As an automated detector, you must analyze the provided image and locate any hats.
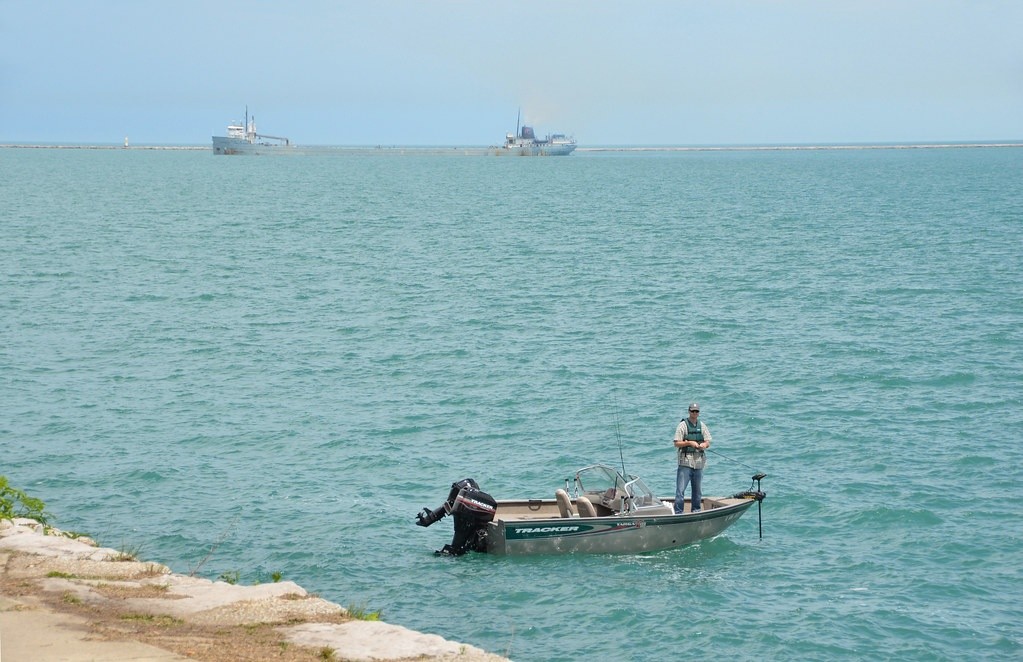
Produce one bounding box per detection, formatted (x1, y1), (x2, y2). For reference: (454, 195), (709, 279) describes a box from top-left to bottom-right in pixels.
(688, 402), (700, 412)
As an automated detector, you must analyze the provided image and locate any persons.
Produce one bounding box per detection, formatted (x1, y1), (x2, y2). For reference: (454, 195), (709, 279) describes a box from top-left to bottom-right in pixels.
(673, 403), (712, 516)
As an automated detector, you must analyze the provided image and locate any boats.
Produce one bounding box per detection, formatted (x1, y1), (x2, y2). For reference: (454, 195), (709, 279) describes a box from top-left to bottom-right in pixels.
(210, 103), (292, 160)
(459, 105), (577, 158)
(413, 460), (768, 565)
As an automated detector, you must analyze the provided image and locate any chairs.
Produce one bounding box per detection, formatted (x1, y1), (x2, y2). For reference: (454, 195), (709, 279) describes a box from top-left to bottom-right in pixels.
(556, 488), (574, 518)
(576, 497), (596, 518)
